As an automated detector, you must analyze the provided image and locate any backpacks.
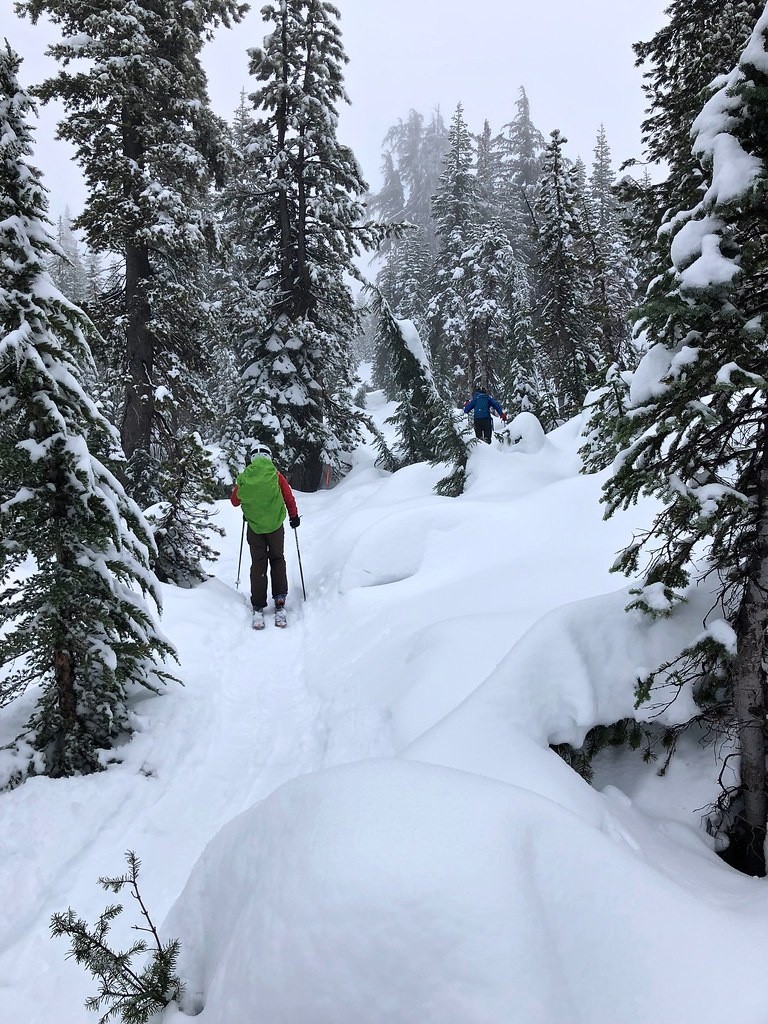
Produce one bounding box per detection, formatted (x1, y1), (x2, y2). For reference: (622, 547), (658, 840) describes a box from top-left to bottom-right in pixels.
(237, 456), (286, 534)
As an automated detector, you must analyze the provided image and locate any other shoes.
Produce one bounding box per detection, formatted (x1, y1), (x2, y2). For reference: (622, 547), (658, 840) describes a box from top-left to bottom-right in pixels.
(253, 604), (263, 613)
(275, 594), (285, 609)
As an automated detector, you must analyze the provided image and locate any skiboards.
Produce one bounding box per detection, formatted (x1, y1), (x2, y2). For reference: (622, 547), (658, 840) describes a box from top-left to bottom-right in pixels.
(252, 608), (286, 631)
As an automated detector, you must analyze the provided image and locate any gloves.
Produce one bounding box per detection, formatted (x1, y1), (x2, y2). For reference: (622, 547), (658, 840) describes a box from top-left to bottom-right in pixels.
(465, 400), (470, 406)
(289, 514), (300, 528)
(500, 414), (506, 421)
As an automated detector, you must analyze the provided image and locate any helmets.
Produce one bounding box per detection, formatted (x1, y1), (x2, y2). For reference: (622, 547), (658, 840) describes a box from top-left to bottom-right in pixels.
(476, 387), (486, 393)
(250, 445), (272, 463)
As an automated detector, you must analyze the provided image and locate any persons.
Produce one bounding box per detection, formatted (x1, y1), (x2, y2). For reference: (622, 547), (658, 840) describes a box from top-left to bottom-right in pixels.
(231, 444), (300, 629)
(464, 387), (506, 444)
